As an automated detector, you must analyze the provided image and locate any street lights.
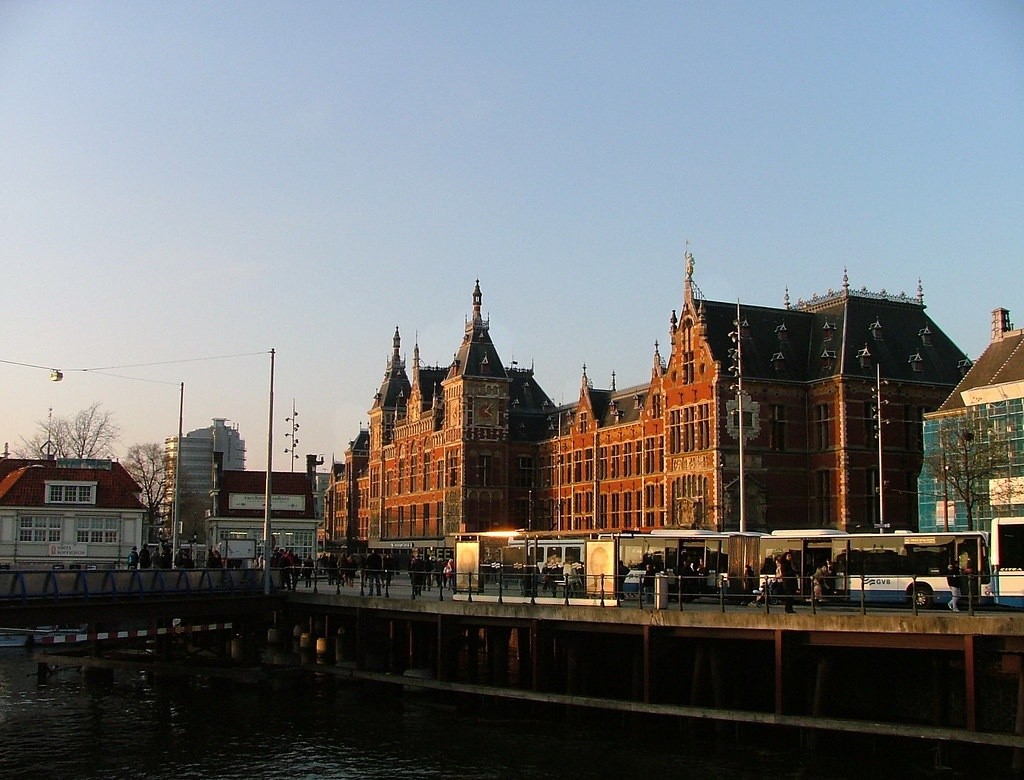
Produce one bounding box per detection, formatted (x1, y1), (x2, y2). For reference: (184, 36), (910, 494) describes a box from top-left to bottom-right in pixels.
(872, 364), (891, 534)
(284, 398), (299, 471)
(728, 298), (749, 533)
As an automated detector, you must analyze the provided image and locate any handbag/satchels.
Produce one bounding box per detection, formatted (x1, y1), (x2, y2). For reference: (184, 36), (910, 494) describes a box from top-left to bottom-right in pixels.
(446, 563), (452, 577)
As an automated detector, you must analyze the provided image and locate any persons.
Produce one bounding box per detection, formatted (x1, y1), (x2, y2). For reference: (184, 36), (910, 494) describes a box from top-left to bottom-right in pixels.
(526, 556), (540, 594)
(678, 558), (704, 603)
(947, 562), (962, 612)
(964, 561), (978, 595)
(484, 552), (501, 582)
(814, 559), (833, 599)
(685, 251), (695, 278)
(227, 559), (243, 568)
(741, 565), (756, 604)
(776, 554), (804, 613)
(563, 557), (578, 598)
(580, 564), (584, 586)
(620, 560), (630, 601)
(634, 564), (659, 604)
(408, 555), (454, 596)
(542, 563), (560, 597)
(270, 548), (395, 596)
(259, 557), (263, 569)
(207, 549), (222, 568)
(128, 543), (195, 569)
(638, 563), (644, 569)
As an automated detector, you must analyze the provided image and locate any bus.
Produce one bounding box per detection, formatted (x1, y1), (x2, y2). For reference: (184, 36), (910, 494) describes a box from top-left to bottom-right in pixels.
(990, 517), (1024, 608)
(598, 529), (772, 599)
(759, 529), (993, 610)
(508, 540), (585, 585)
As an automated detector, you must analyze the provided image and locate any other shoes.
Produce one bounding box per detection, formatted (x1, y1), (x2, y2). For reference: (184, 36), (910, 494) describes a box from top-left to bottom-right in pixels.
(784, 609), (796, 613)
(953, 609), (960, 612)
(948, 603), (952, 609)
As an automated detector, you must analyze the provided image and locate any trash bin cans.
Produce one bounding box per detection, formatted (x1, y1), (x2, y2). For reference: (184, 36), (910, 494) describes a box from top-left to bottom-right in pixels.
(654, 570), (669, 610)
